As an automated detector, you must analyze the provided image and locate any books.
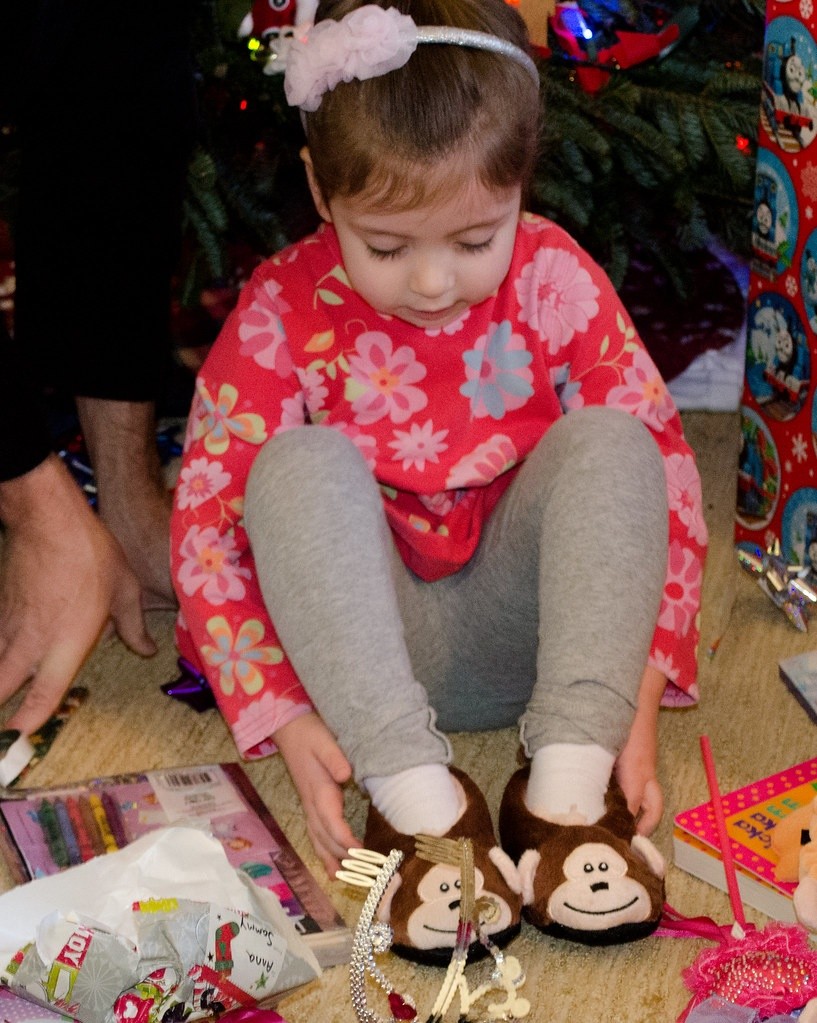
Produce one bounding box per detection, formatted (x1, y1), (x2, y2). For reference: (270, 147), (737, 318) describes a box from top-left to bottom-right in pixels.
(671, 759), (817, 921)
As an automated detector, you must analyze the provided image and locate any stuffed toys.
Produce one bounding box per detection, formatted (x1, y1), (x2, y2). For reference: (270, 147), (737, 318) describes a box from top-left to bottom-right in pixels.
(773, 796), (817, 931)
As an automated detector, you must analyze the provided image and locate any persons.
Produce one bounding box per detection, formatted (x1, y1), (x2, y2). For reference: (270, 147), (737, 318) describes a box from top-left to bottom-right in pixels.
(171, 0), (708, 968)
(0, 0), (198, 734)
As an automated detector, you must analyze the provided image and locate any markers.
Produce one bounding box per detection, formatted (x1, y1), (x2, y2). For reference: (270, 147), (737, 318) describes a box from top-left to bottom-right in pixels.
(36, 790), (126, 870)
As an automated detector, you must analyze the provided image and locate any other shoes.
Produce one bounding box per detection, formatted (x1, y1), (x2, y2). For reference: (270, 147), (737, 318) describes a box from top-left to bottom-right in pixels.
(364, 764), (521, 969)
(498, 757), (666, 946)
